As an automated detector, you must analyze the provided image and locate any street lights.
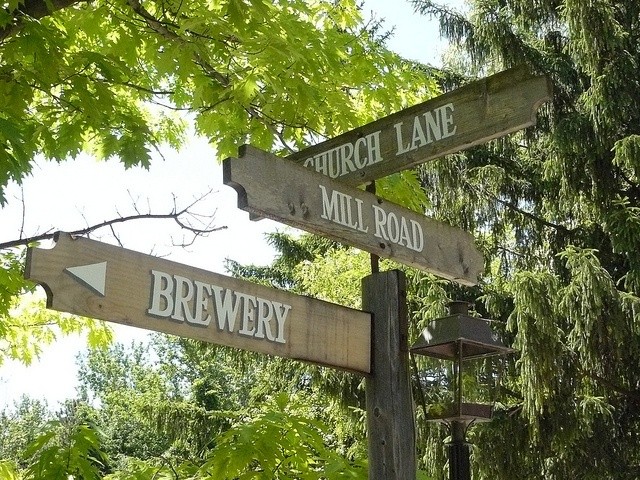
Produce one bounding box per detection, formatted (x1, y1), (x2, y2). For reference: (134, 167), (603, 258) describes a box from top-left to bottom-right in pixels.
(410, 302), (518, 480)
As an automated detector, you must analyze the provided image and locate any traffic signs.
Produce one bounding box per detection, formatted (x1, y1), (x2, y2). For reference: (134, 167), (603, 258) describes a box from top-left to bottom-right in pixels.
(223, 144), (484, 288)
(249, 64), (553, 221)
(25, 231), (371, 374)
